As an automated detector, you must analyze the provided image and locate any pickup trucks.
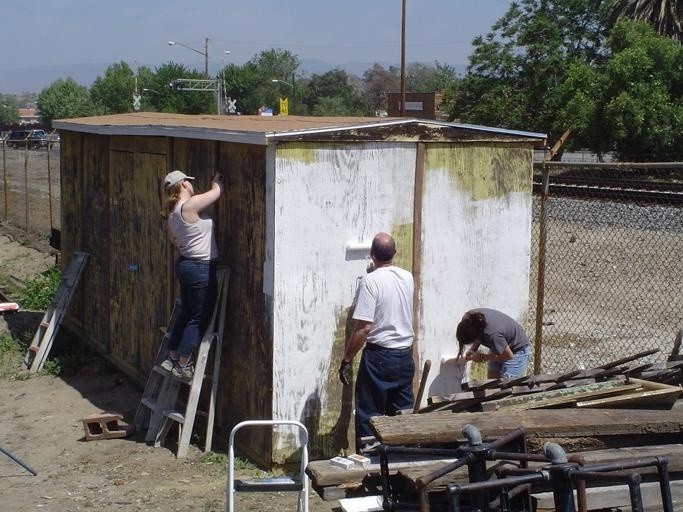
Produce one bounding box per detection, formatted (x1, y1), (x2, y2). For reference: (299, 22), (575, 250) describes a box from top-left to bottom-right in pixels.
(10, 130), (60, 150)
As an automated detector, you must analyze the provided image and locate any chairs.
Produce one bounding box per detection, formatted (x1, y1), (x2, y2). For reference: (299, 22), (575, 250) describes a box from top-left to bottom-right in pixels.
(226, 419), (309, 512)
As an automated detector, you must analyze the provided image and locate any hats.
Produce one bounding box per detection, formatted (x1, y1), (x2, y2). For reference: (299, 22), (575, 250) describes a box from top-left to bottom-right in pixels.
(163, 171), (195, 191)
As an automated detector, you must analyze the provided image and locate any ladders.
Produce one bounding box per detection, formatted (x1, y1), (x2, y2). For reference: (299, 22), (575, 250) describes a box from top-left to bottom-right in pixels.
(21, 251), (89, 374)
(132, 268), (231, 459)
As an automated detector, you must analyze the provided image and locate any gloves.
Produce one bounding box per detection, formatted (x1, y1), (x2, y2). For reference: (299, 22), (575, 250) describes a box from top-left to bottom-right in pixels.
(340, 359), (350, 386)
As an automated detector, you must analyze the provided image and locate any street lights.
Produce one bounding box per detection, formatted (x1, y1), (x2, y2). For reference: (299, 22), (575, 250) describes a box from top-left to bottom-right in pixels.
(143, 88), (160, 112)
(168, 37), (209, 76)
(272, 73), (296, 116)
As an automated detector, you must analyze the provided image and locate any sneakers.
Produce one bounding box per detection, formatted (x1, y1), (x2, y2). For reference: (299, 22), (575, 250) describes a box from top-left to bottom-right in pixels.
(161, 357), (207, 377)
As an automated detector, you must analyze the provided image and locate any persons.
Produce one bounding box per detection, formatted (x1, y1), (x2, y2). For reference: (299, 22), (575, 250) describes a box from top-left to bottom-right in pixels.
(159, 170), (224, 382)
(337, 233), (414, 453)
(455, 308), (530, 380)
